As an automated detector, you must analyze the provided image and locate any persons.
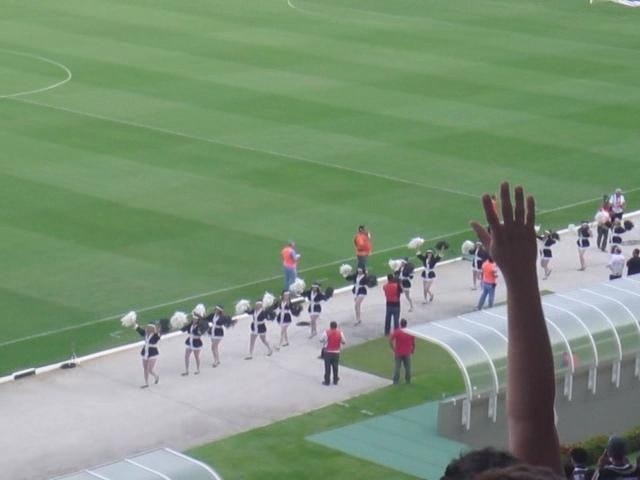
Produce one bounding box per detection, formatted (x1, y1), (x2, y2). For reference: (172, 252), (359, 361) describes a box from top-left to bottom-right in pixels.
(131, 320), (160, 389)
(243, 300), (273, 359)
(319, 321), (346, 385)
(206, 305), (231, 368)
(352, 225), (370, 269)
(298, 282), (329, 338)
(440, 178), (568, 480)
(380, 187), (640, 337)
(179, 315), (201, 375)
(591, 433), (635, 480)
(270, 289), (303, 350)
(346, 265), (370, 326)
(567, 447), (594, 480)
(281, 240), (301, 289)
(387, 319), (416, 385)
